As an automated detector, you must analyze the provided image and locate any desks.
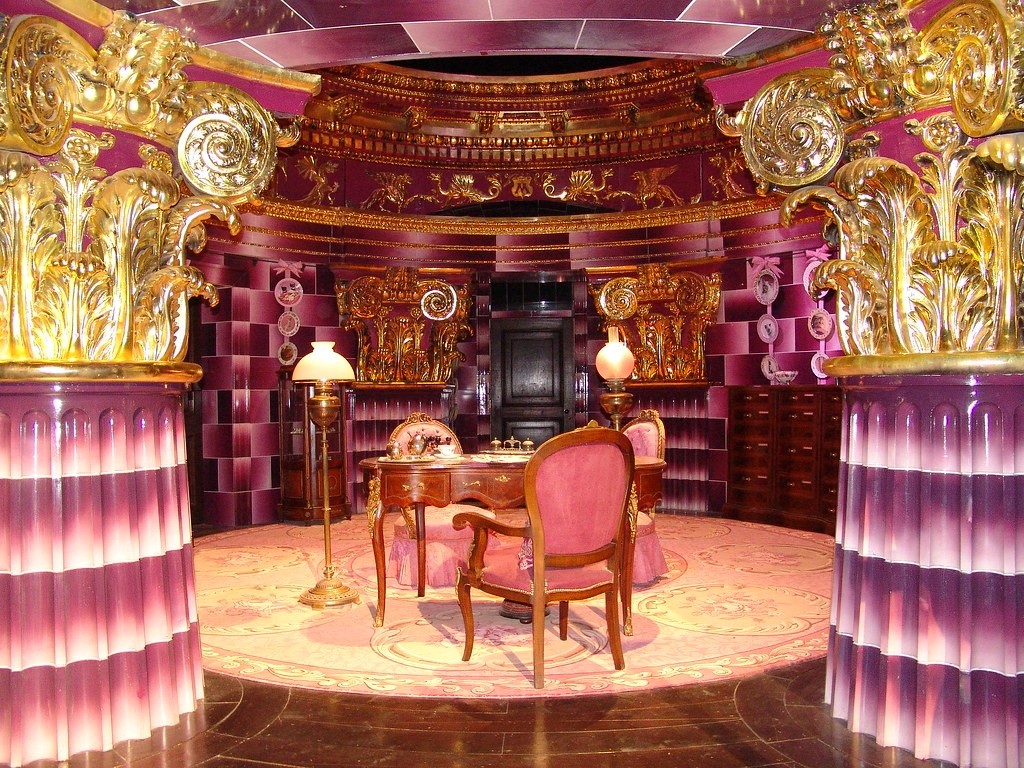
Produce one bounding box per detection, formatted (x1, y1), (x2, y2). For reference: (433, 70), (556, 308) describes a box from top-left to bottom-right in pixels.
(358, 453), (669, 637)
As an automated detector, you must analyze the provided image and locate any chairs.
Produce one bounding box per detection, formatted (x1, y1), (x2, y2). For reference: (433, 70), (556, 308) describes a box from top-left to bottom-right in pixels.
(451, 420), (634, 690)
(385, 412), (502, 588)
(619, 409), (668, 585)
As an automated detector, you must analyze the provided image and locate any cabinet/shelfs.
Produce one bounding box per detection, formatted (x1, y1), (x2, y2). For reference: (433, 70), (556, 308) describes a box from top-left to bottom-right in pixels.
(729, 384), (843, 538)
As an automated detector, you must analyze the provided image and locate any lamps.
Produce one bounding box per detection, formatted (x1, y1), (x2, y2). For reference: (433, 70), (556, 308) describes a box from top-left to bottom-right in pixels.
(596, 326), (634, 432)
(292, 340), (361, 612)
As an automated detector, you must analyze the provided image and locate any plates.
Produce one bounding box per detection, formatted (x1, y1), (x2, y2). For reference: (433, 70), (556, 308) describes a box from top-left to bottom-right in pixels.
(434, 454), (460, 459)
(757, 314), (778, 343)
(761, 355), (779, 380)
(803, 261), (829, 299)
(811, 353), (832, 379)
(808, 308), (832, 339)
(278, 342), (298, 365)
(274, 278), (304, 307)
(278, 311), (300, 337)
(752, 269), (779, 305)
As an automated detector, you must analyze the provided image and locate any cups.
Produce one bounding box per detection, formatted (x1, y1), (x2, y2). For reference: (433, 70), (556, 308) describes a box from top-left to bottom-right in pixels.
(438, 445), (456, 456)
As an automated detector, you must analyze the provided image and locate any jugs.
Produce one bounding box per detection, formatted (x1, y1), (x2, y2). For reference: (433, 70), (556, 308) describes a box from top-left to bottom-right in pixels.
(406, 432), (428, 457)
(386, 442), (404, 460)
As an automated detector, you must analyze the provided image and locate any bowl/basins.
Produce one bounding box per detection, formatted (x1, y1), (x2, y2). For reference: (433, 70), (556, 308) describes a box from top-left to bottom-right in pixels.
(773, 370), (798, 384)
